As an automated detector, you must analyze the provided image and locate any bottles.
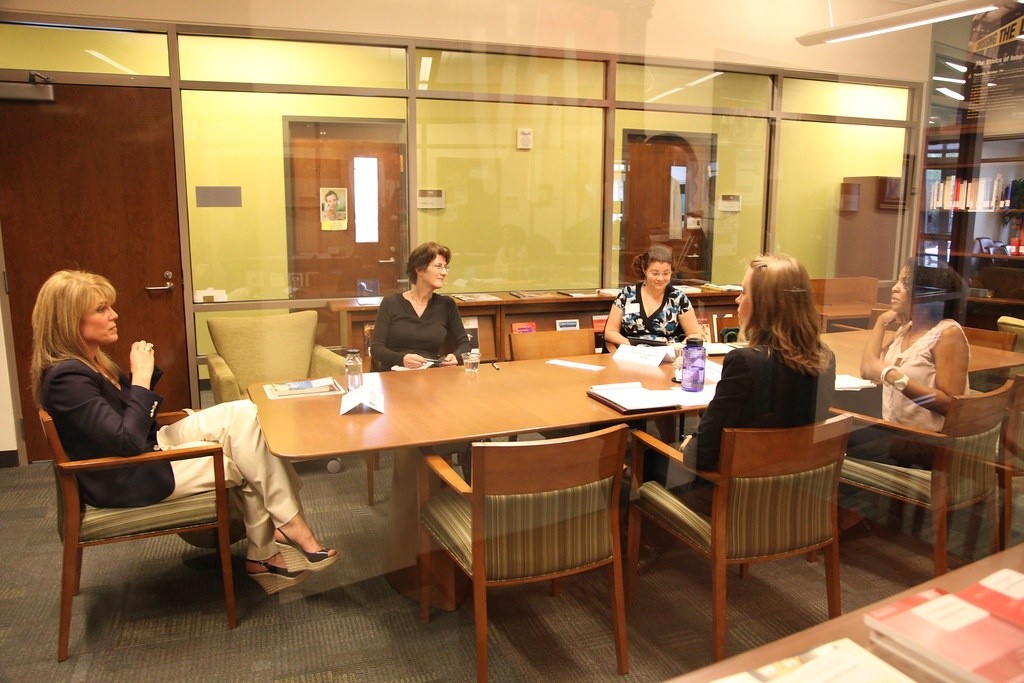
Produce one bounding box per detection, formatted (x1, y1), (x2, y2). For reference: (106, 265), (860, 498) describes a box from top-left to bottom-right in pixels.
(344, 349), (363, 393)
(682, 337), (706, 391)
(327, 458), (342, 473)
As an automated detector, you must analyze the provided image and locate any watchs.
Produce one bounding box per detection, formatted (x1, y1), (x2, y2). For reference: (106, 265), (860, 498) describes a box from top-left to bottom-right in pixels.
(893, 375), (909, 391)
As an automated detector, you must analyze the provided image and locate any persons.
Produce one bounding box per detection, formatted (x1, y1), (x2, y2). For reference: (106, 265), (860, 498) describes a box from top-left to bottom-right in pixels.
(368, 241), (492, 486)
(602, 245), (705, 444)
(323, 190), (346, 221)
(838, 258), (972, 543)
(28, 271), (339, 595)
(636, 255), (835, 579)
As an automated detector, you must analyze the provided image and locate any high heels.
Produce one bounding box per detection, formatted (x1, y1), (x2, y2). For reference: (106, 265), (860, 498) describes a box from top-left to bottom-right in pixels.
(274, 528), (340, 573)
(244, 558), (313, 595)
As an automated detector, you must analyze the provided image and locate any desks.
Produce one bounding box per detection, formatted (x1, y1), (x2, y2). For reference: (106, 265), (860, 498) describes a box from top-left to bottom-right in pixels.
(247, 332), (1024, 629)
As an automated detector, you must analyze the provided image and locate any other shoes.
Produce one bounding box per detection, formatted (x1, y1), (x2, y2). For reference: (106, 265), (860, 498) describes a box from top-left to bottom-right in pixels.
(635, 526), (675, 575)
(838, 516), (874, 540)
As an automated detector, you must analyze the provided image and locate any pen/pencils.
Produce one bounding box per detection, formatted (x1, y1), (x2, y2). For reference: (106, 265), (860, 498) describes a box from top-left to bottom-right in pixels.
(491, 360), (499, 370)
(884, 319), (896, 326)
(424, 359), (448, 362)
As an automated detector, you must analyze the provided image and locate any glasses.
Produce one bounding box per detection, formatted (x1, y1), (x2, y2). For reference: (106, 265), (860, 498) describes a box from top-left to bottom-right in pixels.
(427, 263), (448, 270)
(646, 271), (673, 276)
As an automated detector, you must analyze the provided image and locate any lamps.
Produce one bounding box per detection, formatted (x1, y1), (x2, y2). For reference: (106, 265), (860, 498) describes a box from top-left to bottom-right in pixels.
(794, 0), (1003, 47)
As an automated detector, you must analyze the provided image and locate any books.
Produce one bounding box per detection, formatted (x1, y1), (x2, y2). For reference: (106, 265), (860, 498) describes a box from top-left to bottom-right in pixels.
(926, 171), (1009, 212)
(587, 390), (682, 415)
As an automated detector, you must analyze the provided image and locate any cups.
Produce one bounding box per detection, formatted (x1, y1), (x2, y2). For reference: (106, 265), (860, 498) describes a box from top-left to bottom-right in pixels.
(675, 347), (684, 381)
(461, 353), (481, 372)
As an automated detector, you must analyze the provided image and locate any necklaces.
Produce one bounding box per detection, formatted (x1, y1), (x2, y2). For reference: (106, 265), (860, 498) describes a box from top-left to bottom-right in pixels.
(410, 290), (428, 307)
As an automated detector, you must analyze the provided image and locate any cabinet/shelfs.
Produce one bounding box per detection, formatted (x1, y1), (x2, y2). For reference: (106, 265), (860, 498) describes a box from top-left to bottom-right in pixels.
(914, 155), (1024, 296)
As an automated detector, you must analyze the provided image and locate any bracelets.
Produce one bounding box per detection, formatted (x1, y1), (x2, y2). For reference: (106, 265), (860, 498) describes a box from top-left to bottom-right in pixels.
(880, 367), (896, 386)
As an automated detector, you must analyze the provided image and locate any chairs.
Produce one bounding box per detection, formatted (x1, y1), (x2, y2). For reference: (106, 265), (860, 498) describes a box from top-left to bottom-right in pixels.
(827, 375), (1024, 576)
(206, 310), (347, 474)
(509, 327), (596, 440)
(998, 317), (1024, 460)
(625, 410), (855, 663)
(961, 326), (1017, 379)
(419, 423), (630, 683)
(38, 407), (237, 661)
